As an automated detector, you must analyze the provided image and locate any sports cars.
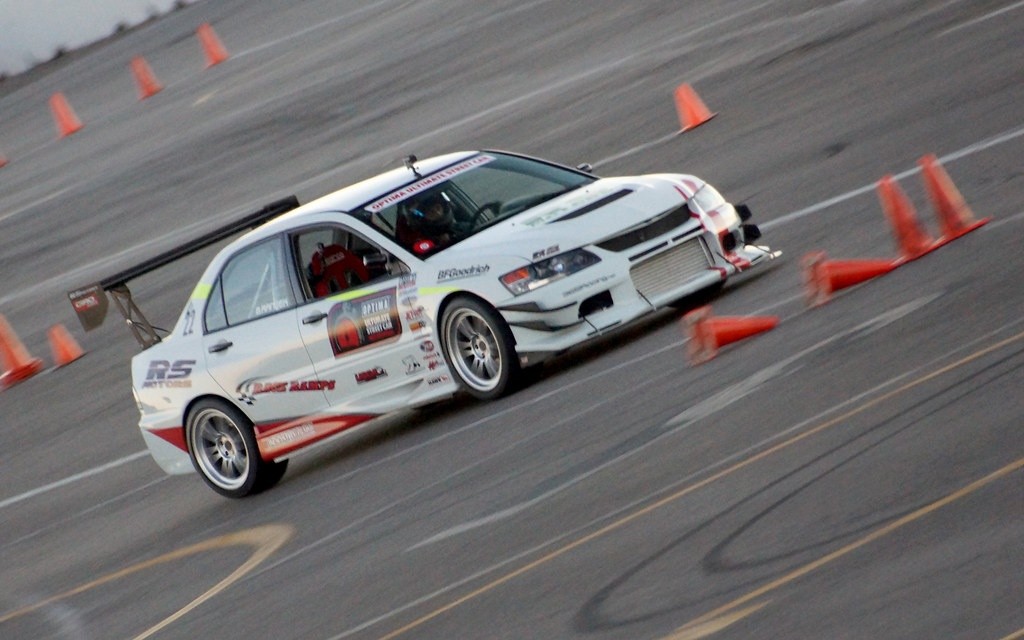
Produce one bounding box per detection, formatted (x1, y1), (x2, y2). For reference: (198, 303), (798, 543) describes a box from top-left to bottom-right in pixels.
(94, 147), (778, 503)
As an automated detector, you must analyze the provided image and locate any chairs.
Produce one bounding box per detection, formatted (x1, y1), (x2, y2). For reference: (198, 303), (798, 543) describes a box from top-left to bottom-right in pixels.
(306, 243), (372, 300)
(392, 201), (435, 254)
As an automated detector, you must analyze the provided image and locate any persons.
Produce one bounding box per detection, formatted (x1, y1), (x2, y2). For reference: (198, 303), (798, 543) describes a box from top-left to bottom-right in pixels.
(402, 191), (476, 254)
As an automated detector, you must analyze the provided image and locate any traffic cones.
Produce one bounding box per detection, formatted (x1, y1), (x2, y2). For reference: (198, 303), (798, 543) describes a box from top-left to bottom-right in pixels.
(199, 20), (228, 69)
(871, 178), (942, 270)
(672, 83), (717, 139)
(0, 315), (47, 384)
(912, 150), (989, 245)
(44, 325), (89, 372)
(49, 93), (84, 141)
(132, 59), (165, 104)
(682, 305), (781, 367)
(801, 247), (895, 300)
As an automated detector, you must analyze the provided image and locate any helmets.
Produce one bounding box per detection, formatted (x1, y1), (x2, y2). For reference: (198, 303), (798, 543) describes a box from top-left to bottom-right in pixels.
(402, 189), (453, 235)
